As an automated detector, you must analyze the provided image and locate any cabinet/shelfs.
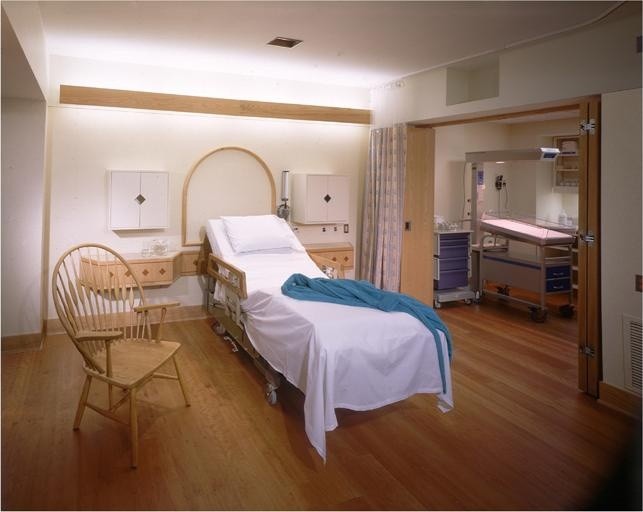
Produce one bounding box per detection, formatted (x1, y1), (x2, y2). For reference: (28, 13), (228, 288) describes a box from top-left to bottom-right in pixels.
(292, 173), (351, 227)
(105, 169), (171, 230)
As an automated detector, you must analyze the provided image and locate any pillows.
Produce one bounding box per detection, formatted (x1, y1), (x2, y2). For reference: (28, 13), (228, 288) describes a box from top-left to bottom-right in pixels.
(222, 215), (299, 253)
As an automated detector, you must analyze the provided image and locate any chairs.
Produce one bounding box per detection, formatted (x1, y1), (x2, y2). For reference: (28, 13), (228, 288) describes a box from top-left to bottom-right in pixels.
(52, 242), (191, 471)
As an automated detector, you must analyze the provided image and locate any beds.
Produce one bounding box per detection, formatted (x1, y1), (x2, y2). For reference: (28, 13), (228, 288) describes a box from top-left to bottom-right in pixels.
(194, 218), (448, 408)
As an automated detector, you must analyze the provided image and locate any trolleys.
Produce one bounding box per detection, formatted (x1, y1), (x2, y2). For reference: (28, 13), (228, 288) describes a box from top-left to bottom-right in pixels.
(433, 208), (576, 322)
